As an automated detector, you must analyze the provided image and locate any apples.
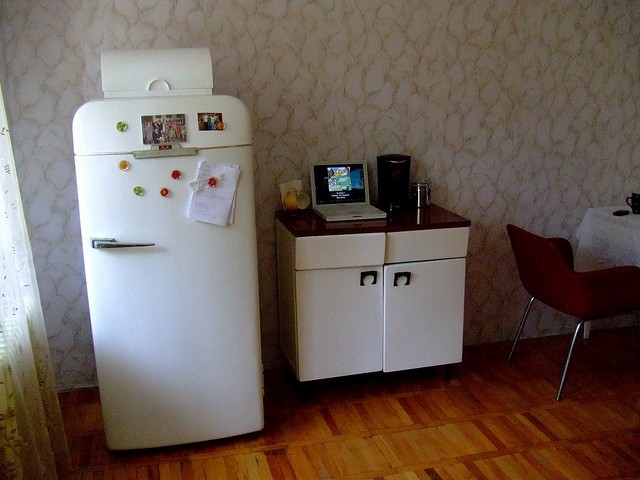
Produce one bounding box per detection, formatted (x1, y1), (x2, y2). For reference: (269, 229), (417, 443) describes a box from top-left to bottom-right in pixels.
(286, 196), (297, 211)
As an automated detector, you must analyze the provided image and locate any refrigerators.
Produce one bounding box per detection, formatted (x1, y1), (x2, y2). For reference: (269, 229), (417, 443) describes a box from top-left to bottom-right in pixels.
(71, 95), (264, 451)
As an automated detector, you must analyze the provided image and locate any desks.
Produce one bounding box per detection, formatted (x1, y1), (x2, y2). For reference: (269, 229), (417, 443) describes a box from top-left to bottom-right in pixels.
(584, 204), (640, 339)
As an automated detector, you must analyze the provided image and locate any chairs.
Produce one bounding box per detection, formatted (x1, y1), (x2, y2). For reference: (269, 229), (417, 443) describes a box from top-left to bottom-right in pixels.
(505, 224), (640, 402)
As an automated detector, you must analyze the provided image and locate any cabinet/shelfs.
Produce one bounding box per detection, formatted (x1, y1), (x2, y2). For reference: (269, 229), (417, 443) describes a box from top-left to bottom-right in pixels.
(274, 202), (471, 400)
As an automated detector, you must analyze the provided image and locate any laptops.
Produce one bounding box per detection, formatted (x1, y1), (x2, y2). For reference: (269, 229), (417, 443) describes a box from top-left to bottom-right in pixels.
(309, 160), (388, 223)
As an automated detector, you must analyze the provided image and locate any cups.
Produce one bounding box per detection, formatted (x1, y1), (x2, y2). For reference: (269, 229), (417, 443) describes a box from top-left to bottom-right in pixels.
(626, 191), (639, 214)
(411, 179), (432, 208)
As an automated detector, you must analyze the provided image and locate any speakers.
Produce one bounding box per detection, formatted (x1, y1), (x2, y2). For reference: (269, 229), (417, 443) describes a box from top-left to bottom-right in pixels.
(377, 155), (412, 215)
